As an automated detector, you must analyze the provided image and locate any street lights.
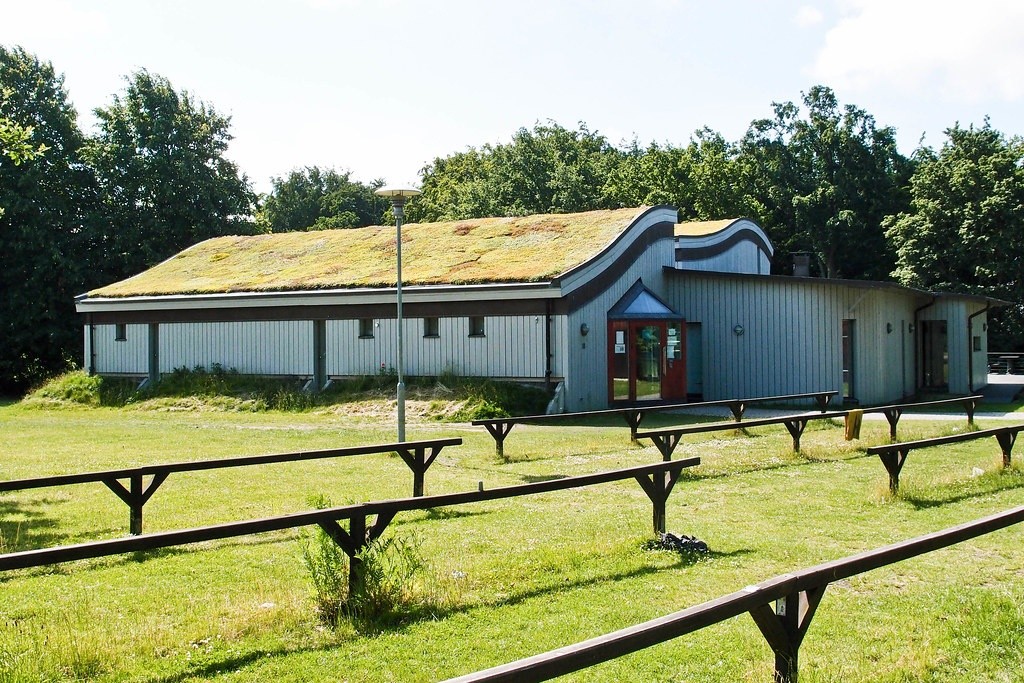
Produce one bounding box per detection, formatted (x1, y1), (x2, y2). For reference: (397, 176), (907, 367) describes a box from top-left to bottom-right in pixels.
(375, 182), (422, 442)
